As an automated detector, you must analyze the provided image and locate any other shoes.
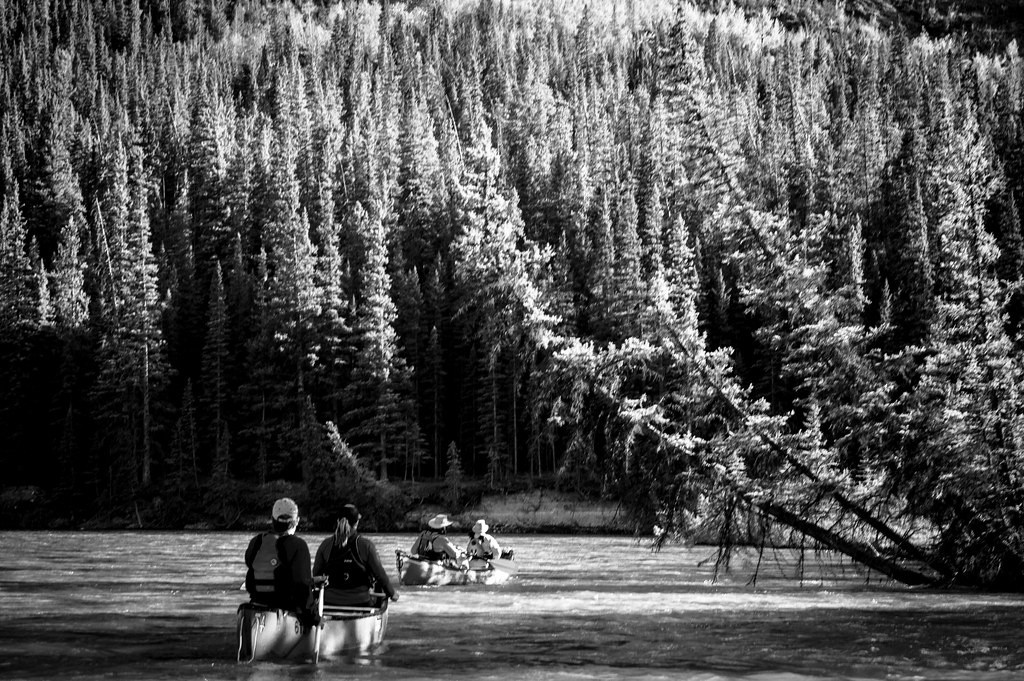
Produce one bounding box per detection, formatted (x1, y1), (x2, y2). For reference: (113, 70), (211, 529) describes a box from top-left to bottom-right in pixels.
(503, 550), (513, 560)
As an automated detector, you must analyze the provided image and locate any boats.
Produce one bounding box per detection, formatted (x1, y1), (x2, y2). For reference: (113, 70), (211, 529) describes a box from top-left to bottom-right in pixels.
(232, 593), (389, 665)
(394, 548), (496, 587)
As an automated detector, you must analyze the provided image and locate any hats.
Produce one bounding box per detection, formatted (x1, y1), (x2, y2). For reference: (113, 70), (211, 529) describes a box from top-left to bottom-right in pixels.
(273, 498), (298, 522)
(339, 503), (359, 527)
(472, 519), (489, 534)
(428, 514), (453, 528)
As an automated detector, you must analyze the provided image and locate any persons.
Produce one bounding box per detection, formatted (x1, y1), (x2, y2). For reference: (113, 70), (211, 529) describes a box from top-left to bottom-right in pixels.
(312, 504), (399, 607)
(467, 519), (512, 560)
(411, 514), (460, 561)
(245, 498), (311, 609)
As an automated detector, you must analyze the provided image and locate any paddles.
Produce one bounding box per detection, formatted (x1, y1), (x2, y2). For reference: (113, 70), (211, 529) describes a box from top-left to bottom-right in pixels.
(463, 553), (517, 574)
(314, 582), (326, 664)
